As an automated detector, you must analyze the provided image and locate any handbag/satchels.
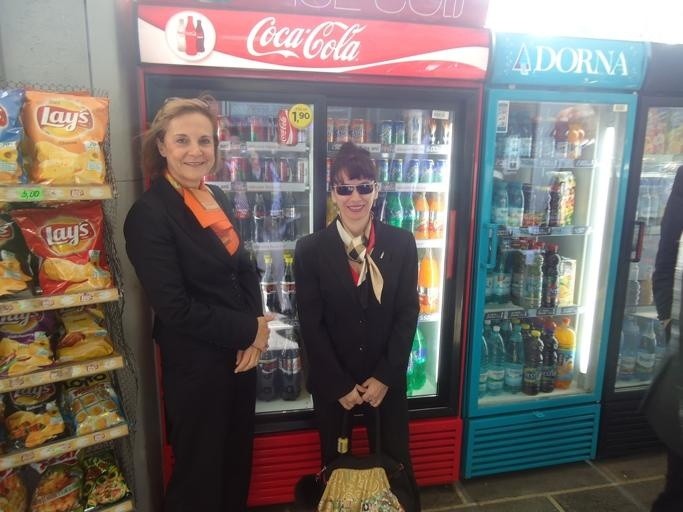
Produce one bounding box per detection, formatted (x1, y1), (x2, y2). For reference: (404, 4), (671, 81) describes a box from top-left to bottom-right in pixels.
(638, 343), (682, 458)
(294, 455), (419, 512)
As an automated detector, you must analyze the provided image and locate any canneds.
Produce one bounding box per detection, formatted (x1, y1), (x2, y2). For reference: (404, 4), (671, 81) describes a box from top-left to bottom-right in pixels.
(203, 108), (309, 186)
(326, 109), (452, 183)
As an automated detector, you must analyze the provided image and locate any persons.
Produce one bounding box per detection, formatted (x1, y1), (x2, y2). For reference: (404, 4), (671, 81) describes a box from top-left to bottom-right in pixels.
(639, 166), (683, 512)
(123, 92), (276, 511)
(292, 141), (421, 512)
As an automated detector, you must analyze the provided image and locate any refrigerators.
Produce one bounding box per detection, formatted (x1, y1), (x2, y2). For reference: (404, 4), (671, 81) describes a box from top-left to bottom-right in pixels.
(460, 31), (648, 482)
(126, 1), (491, 504)
(589, 43), (682, 461)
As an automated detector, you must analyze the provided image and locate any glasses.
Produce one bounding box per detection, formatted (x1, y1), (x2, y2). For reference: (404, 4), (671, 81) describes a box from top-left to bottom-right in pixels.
(161, 96), (210, 109)
(330, 182), (376, 196)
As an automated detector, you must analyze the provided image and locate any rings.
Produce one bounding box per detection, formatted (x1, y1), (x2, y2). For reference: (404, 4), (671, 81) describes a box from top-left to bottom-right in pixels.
(369, 396), (372, 401)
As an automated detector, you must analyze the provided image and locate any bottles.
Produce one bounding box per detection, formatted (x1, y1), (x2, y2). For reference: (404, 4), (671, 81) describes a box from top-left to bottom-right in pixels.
(608, 118), (681, 392)
(475, 102), (599, 399)
(326, 193), (454, 401)
(184, 16), (196, 56)
(201, 189), (313, 405)
(176, 20), (185, 52)
(195, 20), (204, 53)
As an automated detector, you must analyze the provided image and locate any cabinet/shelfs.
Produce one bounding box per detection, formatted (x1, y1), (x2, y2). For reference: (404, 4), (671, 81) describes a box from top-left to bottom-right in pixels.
(0, 185), (134, 512)
